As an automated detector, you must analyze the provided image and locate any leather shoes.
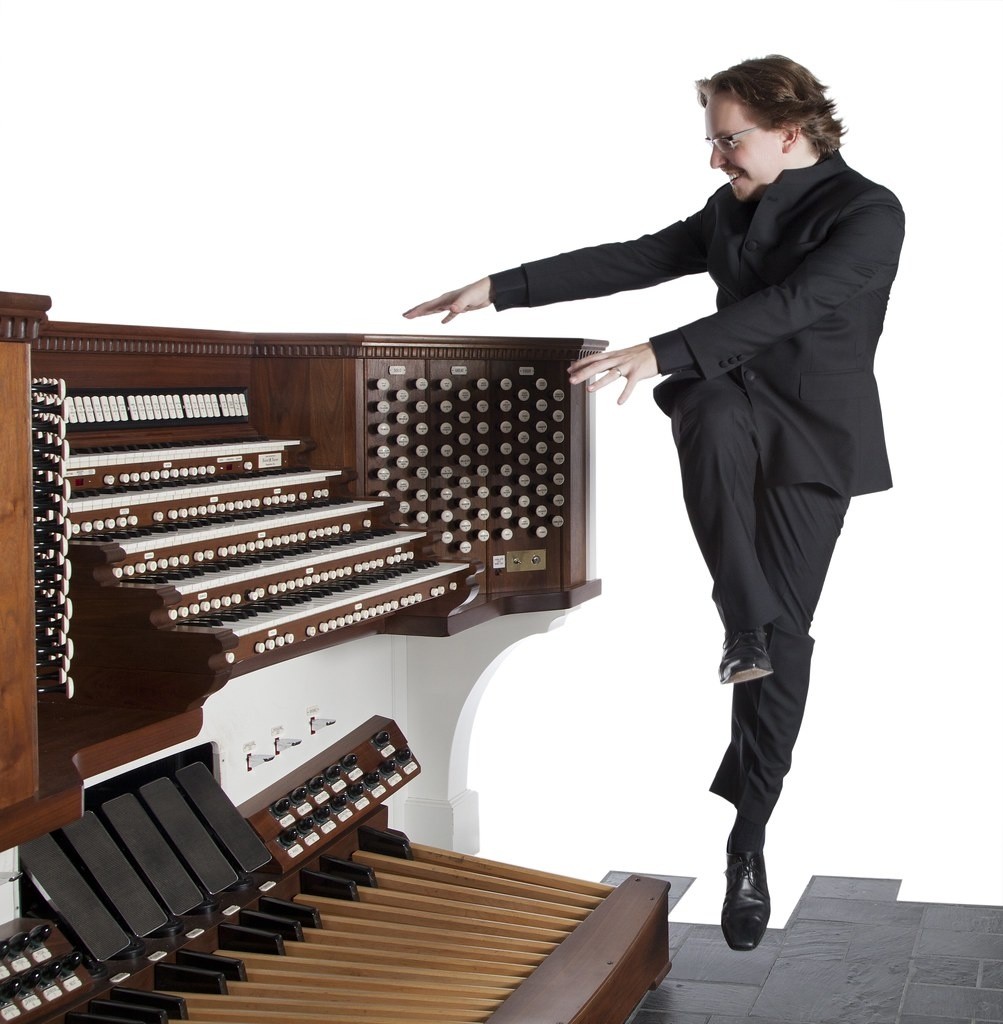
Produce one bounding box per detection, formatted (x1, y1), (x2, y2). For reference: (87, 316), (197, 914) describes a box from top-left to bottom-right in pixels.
(717, 625), (775, 684)
(720, 836), (771, 952)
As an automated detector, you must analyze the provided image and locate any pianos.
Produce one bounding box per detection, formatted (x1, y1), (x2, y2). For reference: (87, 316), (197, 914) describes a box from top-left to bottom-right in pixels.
(0, 290), (672, 1024)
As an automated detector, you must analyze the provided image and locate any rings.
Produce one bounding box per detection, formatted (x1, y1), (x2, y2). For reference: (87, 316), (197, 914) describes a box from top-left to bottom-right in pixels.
(614, 367), (623, 376)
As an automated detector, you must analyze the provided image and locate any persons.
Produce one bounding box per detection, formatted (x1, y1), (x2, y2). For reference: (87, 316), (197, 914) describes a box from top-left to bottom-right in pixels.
(401, 55), (904, 952)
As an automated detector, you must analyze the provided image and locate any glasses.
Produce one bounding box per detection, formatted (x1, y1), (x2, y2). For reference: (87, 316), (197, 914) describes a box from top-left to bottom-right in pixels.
(704, 126), (762, 153)
